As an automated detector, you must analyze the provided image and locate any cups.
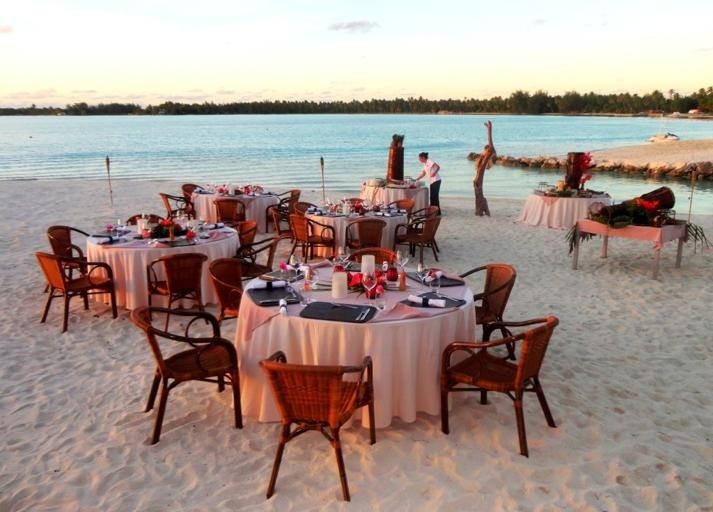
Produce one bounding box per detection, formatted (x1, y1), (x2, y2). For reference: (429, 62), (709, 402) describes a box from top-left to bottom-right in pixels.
(363, 255), (375, 276)
(416, 261), (430, 294)
(332, 273), (345, 300)
(292, 254), (304, 289)
(306, 270), (318, 304)
(338, 245), (351, 270)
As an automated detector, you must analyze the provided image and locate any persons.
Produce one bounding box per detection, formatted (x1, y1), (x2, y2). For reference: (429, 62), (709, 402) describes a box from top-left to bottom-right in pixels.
(416, 151), (442, 216)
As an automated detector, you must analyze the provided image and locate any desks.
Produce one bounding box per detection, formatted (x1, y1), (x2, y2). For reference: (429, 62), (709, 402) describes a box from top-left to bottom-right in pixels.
(571, 219), (687, 280)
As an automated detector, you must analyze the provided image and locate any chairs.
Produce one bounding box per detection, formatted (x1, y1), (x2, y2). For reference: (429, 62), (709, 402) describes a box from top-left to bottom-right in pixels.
(258, 350), (376, 502)
(233, 220), (258, 251)
(146, 252), (209, 334)
(393, 216), (443, 268)
(292, 200), (318, 216)
(181, 182), (205, 199)
(440, 316), (560, 457)
(289, 214), (336, 263)
(235, 237), (279, 277)
(268, 206), (294, 240)
(408, 205), (440, 254)
(34, 250), (117, 330)
(344, 218), (387, 254)
(387, 199), (416, 220)
(213, 198), (246, 225)
(129, 303), (244, 445)
(158, 192), (197, 221)
(453, 262), (517, 361)
(347, 247), (397, 264)
(264, 189), (301, 234)
(347, 196), (365, 204)
(127, 214), (166, 225)
(48, 224), (114, 282)
(207, 257), (244, 325)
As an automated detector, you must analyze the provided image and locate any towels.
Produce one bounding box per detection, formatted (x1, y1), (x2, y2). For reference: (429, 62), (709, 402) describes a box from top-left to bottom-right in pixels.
(406, 293), (447, 312)
(95, 235), (119, 244)
(314, 210), (327, 216)
(248, 190), (261, 198)
(249, 279), (288, 291)
(278, 297), (288, 316)
(376, 211), (390, 216)
(390, 209), (406, 213)
(425, 270), (444, 284)
(204, 222), (227, 230)
(307, 207), (319, 211)
